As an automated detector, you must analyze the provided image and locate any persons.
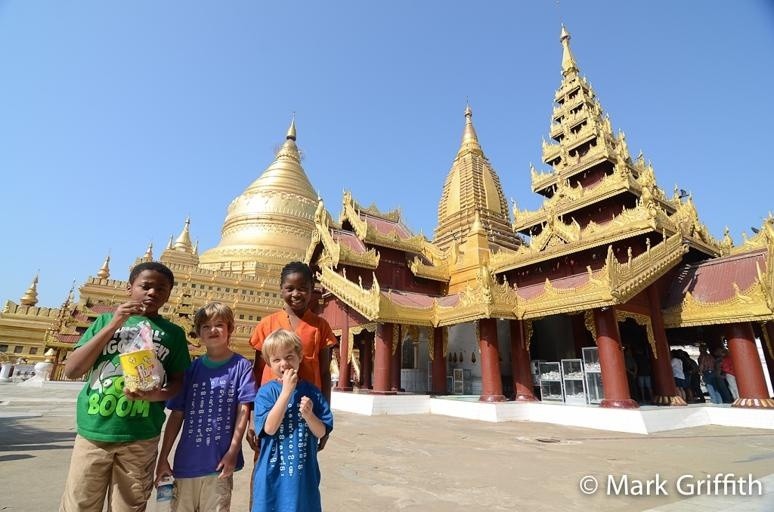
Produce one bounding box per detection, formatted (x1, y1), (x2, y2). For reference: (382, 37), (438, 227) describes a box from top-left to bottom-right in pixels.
(58, 262), (191, 512)
(669, 343), (740, 405)
(622, 346), (654, 404)
(154, 302), (258, 512)
(250, 327), (333, 511)
(246, 261), (340, 512)
